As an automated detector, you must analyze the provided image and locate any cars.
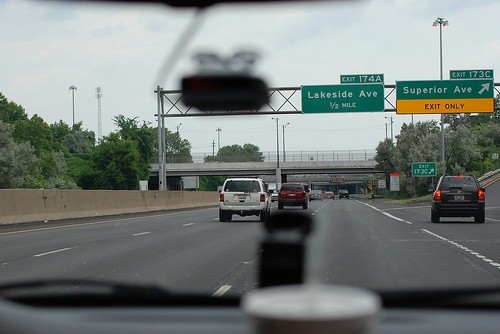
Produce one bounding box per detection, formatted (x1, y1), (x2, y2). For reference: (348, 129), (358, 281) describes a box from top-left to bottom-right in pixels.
(429, 173), (486, 224)
(277, 182), (307, 210)
(323, 191), (335, 200)
(272, 191), (279, 202)
(308, 189), (325, 200)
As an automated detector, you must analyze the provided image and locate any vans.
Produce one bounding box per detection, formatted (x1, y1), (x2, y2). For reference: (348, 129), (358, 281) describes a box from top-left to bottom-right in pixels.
(339, 189), (349, 199)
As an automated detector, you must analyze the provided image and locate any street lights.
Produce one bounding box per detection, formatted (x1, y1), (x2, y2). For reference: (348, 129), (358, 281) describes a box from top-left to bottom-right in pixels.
(384, 115), (394, 142)
(212, 128), (222, 157)
(272, 117), (290, 167)
(68, 84), (79, 126)
(433, 17), (449, 177)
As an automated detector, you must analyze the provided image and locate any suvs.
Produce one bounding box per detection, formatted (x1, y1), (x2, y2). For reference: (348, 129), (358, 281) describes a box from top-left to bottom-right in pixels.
(217, 177), (270, 221)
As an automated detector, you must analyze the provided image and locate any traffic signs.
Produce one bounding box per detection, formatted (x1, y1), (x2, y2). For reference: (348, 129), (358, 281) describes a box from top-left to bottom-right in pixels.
(412, 162), (437, 176)
(301, 69), (495, 113)
